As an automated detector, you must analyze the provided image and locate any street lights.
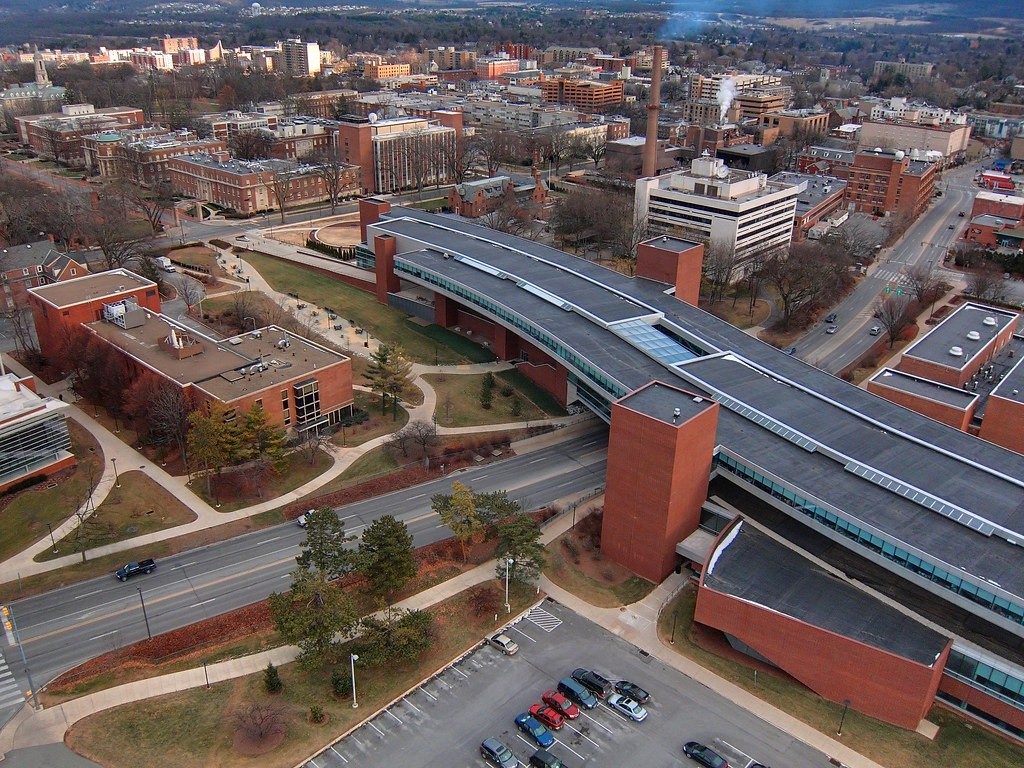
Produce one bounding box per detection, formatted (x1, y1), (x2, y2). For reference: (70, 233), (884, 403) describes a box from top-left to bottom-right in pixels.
(504, 557), (515, 615)
(244, 317), (256, 330)
(188, 289), (204, 321)
(47, 523), (59, 553)
(350, 652), (360, 709)
(111, 457), (122, 488)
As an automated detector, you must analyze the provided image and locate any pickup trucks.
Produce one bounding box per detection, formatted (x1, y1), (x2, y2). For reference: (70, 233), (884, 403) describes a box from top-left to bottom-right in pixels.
(115, 558), (157, 582)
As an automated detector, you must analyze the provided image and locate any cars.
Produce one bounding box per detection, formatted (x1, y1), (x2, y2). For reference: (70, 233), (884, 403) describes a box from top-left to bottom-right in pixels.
(558, 677), (599, 710)
(783, 345), (796, 356)
(616, 680), (651, 703)
(528, 689), (580, 730)
(479, 736), (519, 768)
(529, 749), (570, 768)
(297, 508), (316, 528)
(869, 310), (883, 336)
(484, 631), (520, 656)
(570, 668), (612, 697)
(682, 740), (728, 768)
(825, 313), (838, 335)
(607, 693), (648, 722)
(514, 711), (555, 749)
